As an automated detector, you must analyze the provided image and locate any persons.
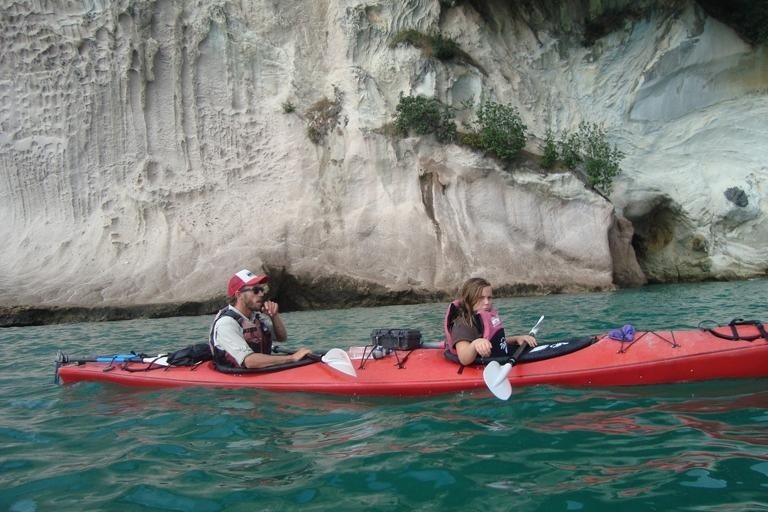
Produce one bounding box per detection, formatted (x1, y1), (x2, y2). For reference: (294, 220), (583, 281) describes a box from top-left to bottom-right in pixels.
(207, 268), (313, 369)
(440, 277), (537, 367)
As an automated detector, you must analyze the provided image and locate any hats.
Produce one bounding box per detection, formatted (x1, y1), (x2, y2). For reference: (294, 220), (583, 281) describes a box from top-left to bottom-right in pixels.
(228, 268), (269, 299)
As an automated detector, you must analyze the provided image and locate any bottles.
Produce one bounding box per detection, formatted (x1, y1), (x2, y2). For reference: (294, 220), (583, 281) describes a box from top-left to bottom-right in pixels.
(348, 345), (392, 358)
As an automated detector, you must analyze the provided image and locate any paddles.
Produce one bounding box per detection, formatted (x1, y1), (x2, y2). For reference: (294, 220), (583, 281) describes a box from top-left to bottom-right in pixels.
(55, 352), (205, 367)
(272, 346), (357, 377)
(483, 316), (543, 400)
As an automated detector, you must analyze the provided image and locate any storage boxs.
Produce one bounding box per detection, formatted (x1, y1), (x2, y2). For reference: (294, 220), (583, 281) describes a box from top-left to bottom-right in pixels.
(370, 328), (421, 353)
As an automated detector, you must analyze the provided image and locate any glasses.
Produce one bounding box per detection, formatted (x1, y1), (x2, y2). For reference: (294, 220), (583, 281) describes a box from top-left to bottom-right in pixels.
(240, 286), (265, 296)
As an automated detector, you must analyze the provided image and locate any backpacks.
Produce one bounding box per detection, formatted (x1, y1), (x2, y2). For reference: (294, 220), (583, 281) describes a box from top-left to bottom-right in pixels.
(165, 343), (214, 368)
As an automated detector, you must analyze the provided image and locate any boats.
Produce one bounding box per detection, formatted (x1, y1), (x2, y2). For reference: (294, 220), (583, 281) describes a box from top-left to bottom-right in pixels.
(53, 317), (768, 397)
